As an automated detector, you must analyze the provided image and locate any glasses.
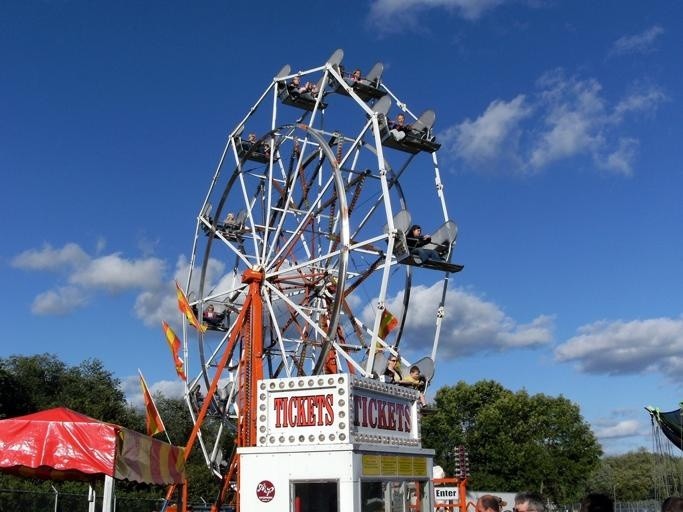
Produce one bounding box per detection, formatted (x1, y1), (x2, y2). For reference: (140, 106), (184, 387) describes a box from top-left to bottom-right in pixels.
(513, 508), (519, 511)
(390, 360), (398, 362)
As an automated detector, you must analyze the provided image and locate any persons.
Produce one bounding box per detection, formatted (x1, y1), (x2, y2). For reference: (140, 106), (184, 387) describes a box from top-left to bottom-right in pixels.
(388, 113), (436, 143)
(204, 304), (226, 332)
(513, 493), (545, 512)
(191, 385), (205, 412)
(386, 117), (406, 142)
(661, 496), (683, 512)
(289, 76), (324, 108)
(580, 492), (614, 512)
(223, 213), (237, 237)
(191, 304), (205, 320)
(477, 495), (500, 512)
(380, 352), (402, 386)
(399, 366), (427, 408)
(406, 225), (446, 264)
(248, 133), (266, 155)
(212, 385), (227, 414)
(350, 70), (377, 89)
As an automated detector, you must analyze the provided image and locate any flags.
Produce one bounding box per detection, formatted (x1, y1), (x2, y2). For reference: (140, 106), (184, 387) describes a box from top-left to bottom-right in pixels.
(175, 281), (208, 333)
(376, 308), (398, 348)
(140, 375), (164, 437)
(162, 320), (187, 381)
